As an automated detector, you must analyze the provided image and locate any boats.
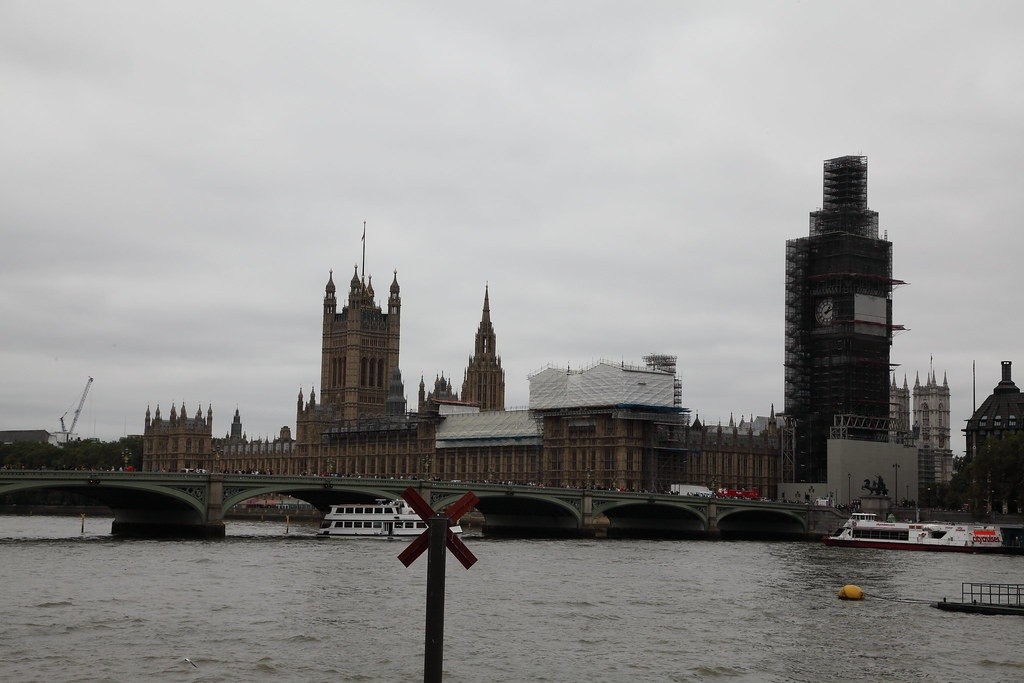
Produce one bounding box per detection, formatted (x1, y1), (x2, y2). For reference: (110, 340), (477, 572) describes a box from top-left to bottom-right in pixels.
(319, 497), (464, 539)
(822, 509), (1012, 554)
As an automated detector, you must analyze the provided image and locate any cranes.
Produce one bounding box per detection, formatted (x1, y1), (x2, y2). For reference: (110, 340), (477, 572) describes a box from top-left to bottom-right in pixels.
(60, 378), (93, 431)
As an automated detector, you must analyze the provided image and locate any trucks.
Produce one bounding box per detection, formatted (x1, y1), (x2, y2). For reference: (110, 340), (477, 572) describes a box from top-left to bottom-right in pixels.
(671, 484), (710, 497)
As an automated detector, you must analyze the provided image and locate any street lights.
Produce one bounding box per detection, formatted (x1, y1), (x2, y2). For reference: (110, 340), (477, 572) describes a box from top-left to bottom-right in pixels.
(422, 453), (432, 481)
(326, 456), (335, 474)
(212, 445), (224, 467)
(847, 473), (852, 506)
(120, 448), (132, 472)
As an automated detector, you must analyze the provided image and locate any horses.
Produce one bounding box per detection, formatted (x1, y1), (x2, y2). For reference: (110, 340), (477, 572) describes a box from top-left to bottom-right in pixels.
(860, 479), (881, 495)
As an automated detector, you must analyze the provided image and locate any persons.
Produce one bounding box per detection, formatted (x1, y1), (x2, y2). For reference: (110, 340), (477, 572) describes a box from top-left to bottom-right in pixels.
(0, 464), (134, 472)
(300, 469), (440, 481)
(484, 478), (799, 504)
(219, 468), (274, 475)
(193, 466), (196, 473)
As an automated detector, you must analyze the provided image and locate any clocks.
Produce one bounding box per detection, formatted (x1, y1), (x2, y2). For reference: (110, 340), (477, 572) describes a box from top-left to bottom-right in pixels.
(815, 295), (838, 326)
